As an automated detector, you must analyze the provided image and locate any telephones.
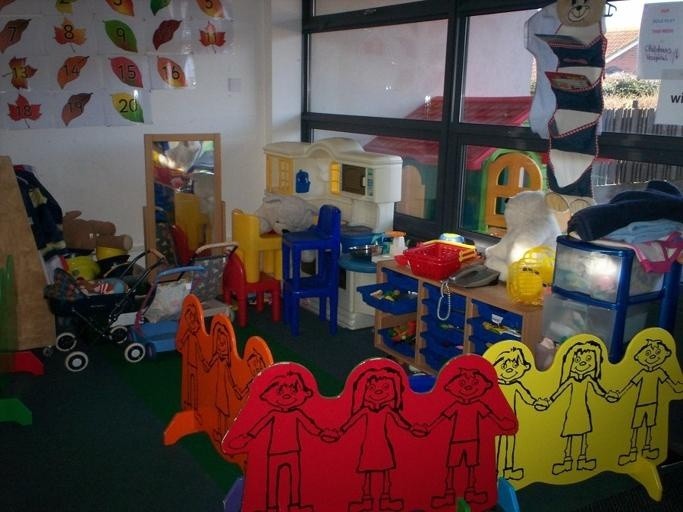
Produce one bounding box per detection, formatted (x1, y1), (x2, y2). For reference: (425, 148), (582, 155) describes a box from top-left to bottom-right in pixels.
(449, 263), (500, 288)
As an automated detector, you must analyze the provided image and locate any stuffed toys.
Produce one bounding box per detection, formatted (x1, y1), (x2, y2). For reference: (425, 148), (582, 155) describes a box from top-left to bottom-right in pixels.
(60, 209), (133, 251)
(252, 193), (320, 235)
(482, 190), (561, 282)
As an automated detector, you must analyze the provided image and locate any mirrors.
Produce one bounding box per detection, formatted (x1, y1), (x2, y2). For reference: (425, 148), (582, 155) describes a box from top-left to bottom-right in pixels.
(143, 134), (222, 281)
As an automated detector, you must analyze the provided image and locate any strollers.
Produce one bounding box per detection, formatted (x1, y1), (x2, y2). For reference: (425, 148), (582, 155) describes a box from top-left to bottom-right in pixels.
(42, 242), (239, 375)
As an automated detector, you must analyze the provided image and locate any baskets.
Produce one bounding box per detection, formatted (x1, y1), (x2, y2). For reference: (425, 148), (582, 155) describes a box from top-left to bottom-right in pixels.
(403, 240), (477, 281)
(507, 246), (557, 308)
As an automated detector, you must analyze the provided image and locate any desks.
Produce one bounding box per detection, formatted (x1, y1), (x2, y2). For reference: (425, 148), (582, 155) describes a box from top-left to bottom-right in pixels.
(374, 260), (542, 380)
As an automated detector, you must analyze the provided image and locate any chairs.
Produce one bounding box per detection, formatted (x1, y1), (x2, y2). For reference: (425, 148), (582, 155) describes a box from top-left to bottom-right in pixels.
(224, 204), (341, 335)
(172, 191), (210, 265)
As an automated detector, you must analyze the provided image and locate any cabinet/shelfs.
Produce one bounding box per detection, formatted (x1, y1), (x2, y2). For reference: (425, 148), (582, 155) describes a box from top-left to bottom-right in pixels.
(542, 235), (683, 365)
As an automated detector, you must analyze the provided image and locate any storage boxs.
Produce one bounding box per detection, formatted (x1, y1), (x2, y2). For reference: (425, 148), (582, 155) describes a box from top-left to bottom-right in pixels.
(356, 268), (523, 373)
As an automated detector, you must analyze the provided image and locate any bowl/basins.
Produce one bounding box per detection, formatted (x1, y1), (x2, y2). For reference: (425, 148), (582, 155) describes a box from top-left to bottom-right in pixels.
(348, 245), (384, 261)
